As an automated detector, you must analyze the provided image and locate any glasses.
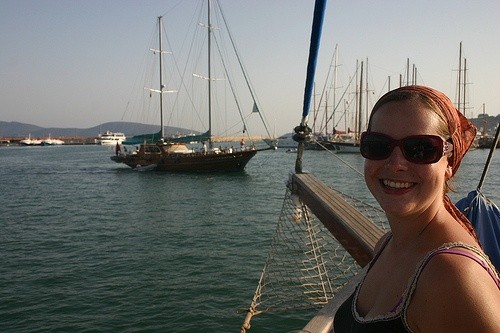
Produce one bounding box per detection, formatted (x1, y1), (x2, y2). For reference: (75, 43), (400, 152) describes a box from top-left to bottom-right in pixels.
(360, 132), (453, 165)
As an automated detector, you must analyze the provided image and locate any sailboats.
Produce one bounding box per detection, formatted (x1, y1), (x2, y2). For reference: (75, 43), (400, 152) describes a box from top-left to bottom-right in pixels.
(110, 0), (279, 173)
(302, 41), (500, 153)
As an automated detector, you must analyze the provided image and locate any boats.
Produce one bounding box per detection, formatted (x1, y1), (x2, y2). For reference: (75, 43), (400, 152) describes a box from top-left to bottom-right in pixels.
(94, 128), (126, 146)
(41, 132), (66, 146)
(18, 134), (44, 147)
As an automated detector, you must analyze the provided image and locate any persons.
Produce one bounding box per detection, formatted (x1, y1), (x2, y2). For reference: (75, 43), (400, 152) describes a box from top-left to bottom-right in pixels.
(334, 85), (500, 333)
(201, 142), (207, 156)
